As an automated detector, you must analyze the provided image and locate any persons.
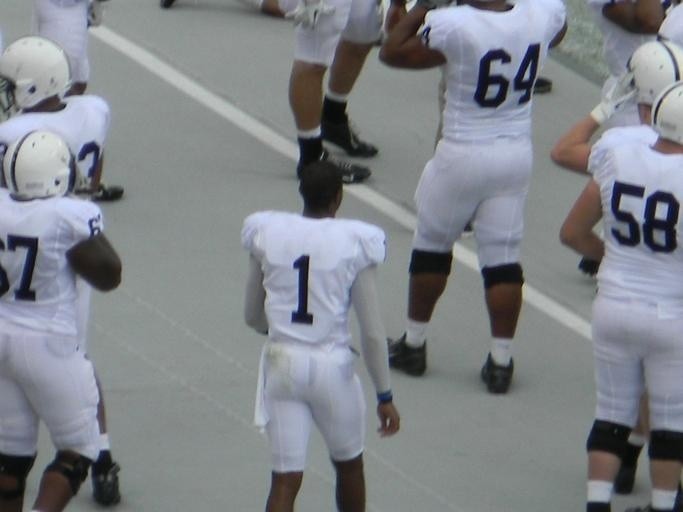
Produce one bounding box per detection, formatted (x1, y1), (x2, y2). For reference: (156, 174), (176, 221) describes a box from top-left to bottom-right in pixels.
(581, 0), (683, 103)
(560, 81), (683, 512)
(1, 36), (121, 506)
(379, 0), (570, 395)
(239, 161), (401, 512)
(551, 38), (683, 498)
(286, 0), (386, 186)
(0, 129), (122, 512)
(28, 0), (125, 203)
(650, 0), (683, 48)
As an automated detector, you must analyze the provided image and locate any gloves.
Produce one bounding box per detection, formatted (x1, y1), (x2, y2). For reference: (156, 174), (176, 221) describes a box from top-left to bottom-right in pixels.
(588, 69), (641, 127)
(287, 2), (338, 30)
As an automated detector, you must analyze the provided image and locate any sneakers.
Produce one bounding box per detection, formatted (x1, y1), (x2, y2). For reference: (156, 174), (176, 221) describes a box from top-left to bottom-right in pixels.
(160, 0), (175, 8)
(90, 184), (125, 202)
(92, 464), (119, 503)
(536, 75), (554, 95)
(614, 462), (641, 495)
(386, 335), (428, 376)
(322, 116), (378, 156)
(297, 149), (371, 185)
(480, 354), (514, 393)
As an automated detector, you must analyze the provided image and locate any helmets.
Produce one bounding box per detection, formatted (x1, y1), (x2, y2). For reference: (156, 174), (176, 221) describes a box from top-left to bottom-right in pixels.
(628, 41), (683, 106)
(3, 131), (78, 199)
(1, 37), (69, 112)
(651, 79), (683, 146)
